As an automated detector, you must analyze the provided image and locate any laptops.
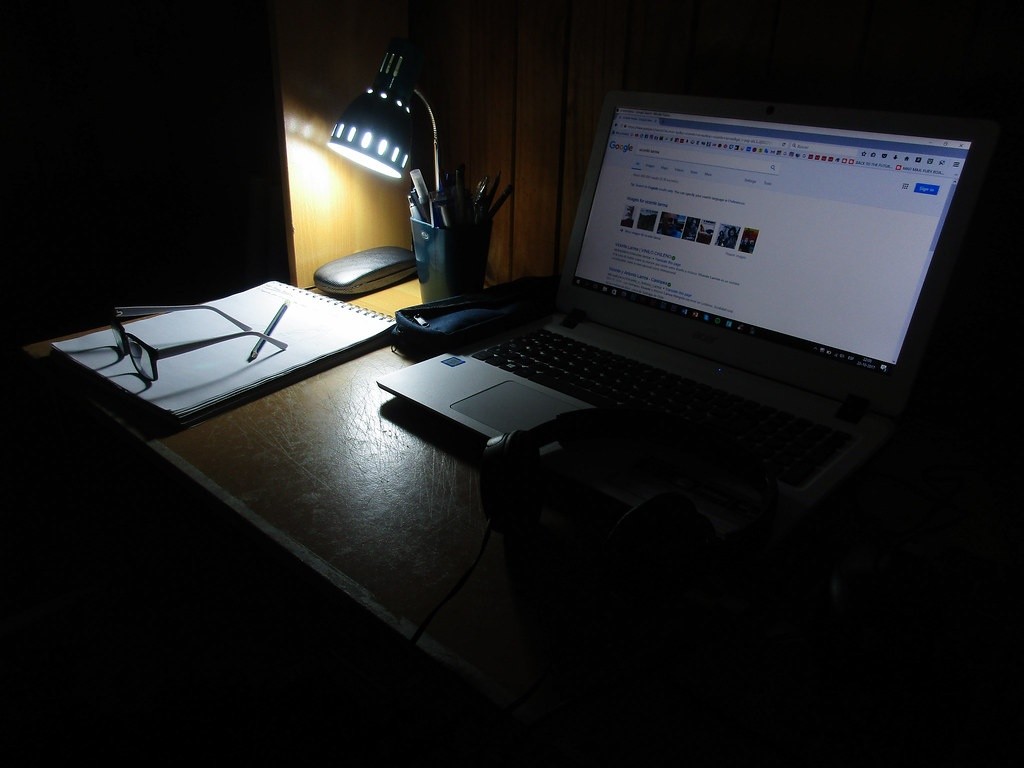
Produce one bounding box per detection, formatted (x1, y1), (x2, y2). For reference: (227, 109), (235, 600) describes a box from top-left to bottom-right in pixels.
(378, 90), (1003, 557)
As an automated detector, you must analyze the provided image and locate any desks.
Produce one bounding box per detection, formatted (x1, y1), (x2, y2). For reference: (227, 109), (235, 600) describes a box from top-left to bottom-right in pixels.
(25, 277), (1023, 768)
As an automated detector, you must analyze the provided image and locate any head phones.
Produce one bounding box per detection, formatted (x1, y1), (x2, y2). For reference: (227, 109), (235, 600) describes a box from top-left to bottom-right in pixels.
(476, 404), (777, 604)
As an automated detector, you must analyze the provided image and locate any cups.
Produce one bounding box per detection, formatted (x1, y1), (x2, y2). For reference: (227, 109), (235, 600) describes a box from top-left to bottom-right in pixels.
(409, 215), (492, 305)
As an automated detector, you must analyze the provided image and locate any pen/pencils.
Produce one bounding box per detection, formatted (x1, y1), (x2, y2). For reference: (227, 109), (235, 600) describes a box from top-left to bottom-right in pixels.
(247, 301), (288, 363)
(402, 167), (514, 232)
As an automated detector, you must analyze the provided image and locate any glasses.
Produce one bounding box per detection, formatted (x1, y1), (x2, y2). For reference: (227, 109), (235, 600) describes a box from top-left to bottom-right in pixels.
(110, 305), (288, 382)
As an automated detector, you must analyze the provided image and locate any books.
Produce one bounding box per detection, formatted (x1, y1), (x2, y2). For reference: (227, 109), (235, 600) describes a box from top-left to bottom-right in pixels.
(52, 277), (399, 428)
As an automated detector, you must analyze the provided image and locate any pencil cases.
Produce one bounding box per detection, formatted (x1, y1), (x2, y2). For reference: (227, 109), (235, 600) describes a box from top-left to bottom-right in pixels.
(392, 289), (522, 361)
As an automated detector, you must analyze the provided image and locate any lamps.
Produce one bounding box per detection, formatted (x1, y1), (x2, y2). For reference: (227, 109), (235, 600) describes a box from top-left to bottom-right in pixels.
(326, 52), (442, 194)
